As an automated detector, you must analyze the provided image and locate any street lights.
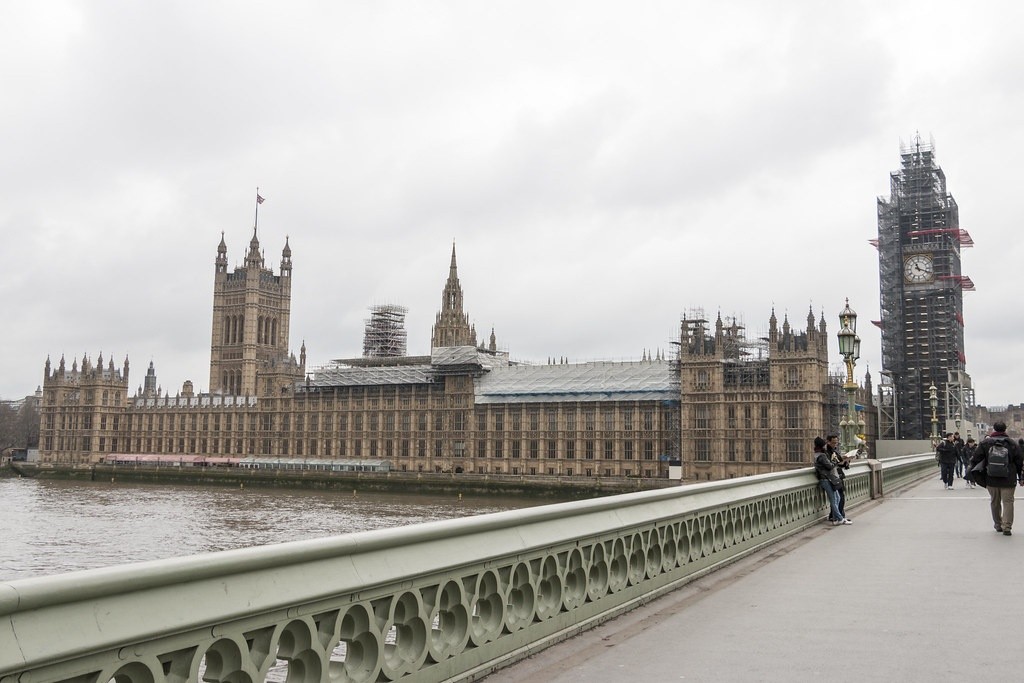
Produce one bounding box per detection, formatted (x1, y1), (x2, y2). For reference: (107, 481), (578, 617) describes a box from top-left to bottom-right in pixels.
(928, 380), (941, 453)
(840, 297), (865, 459)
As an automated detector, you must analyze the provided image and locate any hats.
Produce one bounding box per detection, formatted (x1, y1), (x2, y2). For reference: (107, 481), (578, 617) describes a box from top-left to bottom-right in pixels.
(814, 437), (827, 449)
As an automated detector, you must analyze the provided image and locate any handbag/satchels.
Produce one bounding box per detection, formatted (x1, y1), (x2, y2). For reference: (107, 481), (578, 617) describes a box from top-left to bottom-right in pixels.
(831, 478), (843, 490)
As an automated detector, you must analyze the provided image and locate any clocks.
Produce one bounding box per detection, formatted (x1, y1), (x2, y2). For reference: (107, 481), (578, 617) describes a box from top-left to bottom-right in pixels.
(903, 253), (935, 285)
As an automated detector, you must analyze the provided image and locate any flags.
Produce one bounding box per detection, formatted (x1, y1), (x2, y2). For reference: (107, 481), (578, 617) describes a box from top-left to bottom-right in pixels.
(257, 194), (265, 204)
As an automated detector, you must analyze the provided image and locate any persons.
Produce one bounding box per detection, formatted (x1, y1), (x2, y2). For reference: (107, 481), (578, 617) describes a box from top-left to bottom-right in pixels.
(952, 432), (964, 478)
(962, 439), (977, 489)
(826, 435), (854, 520)
(813, 436), (853, 525)
(936, 433), (963, 490)
(1016, 438), (1024, 486)
(965, 420), (1024, 535)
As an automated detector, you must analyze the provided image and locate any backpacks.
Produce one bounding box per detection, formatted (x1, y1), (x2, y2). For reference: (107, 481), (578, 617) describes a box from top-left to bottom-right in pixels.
(987, 437), (1011, 477)
(935, 441), (955, 467)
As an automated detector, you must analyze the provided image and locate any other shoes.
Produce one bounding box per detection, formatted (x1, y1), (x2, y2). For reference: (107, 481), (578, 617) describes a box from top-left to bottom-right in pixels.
(833, 519), (845, 525)
(944, 483), (948, 489)
(994, 525), (1002, 532)
(965, 483), (969, 489)
(971, 485), (975, 489)
(1003, 531), (1012, 535)
(948, 486), (953, 490)
(841, 518), (853, 525)
